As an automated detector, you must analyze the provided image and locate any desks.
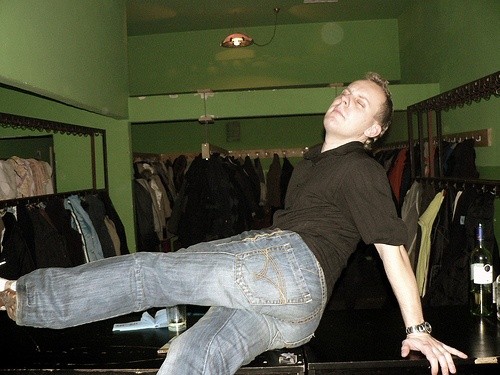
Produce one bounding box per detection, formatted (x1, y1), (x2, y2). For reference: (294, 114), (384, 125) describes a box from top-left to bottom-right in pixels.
(0, 304), (500, 375)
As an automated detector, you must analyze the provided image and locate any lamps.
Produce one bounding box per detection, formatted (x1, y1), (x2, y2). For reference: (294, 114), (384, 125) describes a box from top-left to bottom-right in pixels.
(220, 9), (280, 48)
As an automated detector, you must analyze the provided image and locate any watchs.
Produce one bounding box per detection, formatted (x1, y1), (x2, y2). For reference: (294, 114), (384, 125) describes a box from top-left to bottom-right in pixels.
(406, 322), (432, 334)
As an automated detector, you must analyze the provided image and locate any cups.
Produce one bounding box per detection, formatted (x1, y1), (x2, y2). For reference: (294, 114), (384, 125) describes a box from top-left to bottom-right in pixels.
(166, 304), (187, 332)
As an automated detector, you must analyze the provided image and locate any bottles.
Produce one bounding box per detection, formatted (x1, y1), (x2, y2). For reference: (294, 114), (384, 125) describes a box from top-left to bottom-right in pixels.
(469, 223), (492, 316)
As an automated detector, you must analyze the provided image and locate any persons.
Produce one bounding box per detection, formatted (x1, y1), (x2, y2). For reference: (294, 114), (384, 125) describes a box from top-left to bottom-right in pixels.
(0, 71), (468, 375)
